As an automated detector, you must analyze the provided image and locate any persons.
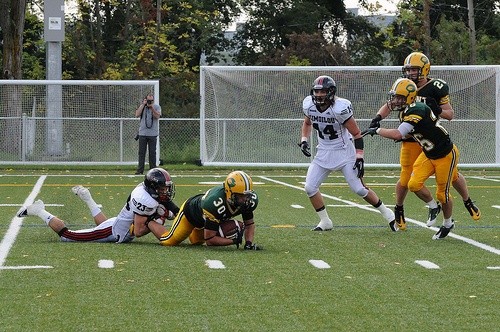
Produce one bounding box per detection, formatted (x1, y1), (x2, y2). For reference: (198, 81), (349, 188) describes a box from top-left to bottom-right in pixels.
(368, 50), (482, 231)
(128, 169), (261, 253)
(17, 166), (178, 243)
(363, 78), (461, 240)
(300, 75), (399, 231)
(135, 93), (162, 176)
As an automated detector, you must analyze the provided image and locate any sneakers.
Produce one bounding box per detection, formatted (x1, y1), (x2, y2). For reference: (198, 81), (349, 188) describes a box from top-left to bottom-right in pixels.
(17, 199), (45, 218)
(394, 205), (406, 230)
(383, 208), (399, 232)
(129, 222), (135, 237)
(426, 201), (441, 227)
(464, 200), (481, 220)
(432, 219), (456, 240)
(310, 219), (333, 231)
(72, 184), (92, 201)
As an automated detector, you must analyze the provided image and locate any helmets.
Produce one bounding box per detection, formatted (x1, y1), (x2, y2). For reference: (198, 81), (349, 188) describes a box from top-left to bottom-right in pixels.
(387, 78), (417, 111)
(224, 170), (254, 205)
(144, 168), (176, 205)
(310, 75), (337, 107)
(401, 51), (430, 86)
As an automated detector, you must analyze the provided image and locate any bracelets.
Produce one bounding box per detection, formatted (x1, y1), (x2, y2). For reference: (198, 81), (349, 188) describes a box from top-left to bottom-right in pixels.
(301, 137), (308, 143)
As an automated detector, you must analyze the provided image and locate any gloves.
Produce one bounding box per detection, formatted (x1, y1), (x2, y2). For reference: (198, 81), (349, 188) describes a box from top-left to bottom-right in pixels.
(428, 97), (442, 115)
(368, 113), (382, 129)
(231, 229), (245, 249)
(297, 140), (311, 157)
(360, 127), (378, 137)
(353, 157), (365, 178)
(244, 241), (261, 251)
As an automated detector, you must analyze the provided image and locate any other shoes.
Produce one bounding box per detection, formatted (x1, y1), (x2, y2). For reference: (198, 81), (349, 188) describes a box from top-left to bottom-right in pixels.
(135, 171), (143, 174)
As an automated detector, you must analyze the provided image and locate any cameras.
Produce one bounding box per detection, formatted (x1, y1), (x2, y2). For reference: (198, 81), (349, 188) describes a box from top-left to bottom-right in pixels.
(146, 99), (152, 104)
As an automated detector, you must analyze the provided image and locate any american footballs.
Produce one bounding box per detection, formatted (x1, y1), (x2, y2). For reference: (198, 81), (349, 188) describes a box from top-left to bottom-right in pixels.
(216, 220), (246, 240)
